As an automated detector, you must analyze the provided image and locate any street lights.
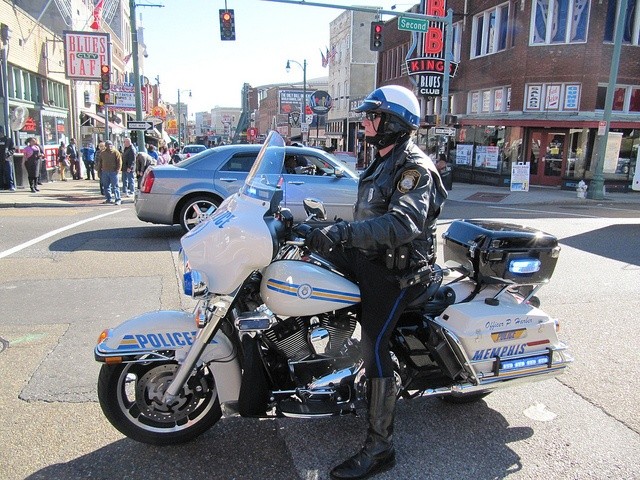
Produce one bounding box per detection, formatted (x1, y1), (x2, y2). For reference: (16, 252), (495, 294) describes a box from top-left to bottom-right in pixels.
(179, 88), (193, 153)
(129, 0), (167, 154)
(286, 59), (309, 145)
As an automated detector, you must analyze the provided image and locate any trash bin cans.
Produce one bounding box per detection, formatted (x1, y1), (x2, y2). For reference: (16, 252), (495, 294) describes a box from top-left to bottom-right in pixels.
(440, 165), (454, 190)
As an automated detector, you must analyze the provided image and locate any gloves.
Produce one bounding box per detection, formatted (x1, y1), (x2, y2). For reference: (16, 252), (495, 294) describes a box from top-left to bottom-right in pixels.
(306, 221), (354, 253)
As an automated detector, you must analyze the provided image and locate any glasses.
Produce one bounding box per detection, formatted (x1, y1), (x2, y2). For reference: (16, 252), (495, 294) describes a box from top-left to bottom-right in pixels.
(366, 111), (382, 120)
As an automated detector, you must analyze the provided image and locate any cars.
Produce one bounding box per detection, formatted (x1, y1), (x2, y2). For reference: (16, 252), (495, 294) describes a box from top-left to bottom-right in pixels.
(134, 144), (361, 231)
(178, 145), (207, 160)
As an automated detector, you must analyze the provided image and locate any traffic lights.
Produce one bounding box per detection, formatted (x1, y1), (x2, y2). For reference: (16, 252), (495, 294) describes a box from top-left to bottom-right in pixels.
(101, 64), (110, 90)
(100, 93), (111, 105)
(219, 9), (235, 40)
(370, 22), (385, 52)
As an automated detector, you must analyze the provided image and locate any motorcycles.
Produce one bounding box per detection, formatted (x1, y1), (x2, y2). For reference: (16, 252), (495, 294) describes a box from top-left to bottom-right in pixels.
(94, 130), (569, 446)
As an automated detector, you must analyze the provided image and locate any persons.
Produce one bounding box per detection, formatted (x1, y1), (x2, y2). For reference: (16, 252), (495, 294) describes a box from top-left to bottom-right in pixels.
(0, 125), (16, 191)
(30, 136), (45, 186)
(303, 85), (450, 478)
(67, 137), (83, 180)
(21, 137), (46, 193)
(97, 140), (123, 204)
(148, 143), (159, 164)
(56, 141), (72, 182)
(146, 141), (191, 165)
(94, 143), (101, 156)
(122, 138), (136, 198)
(434, 153), (448, 178)
(136, 151), (157, 189)
(80, 139), (97, 180)
(330, 145), (337, 155)
(94, 142), (107, 177)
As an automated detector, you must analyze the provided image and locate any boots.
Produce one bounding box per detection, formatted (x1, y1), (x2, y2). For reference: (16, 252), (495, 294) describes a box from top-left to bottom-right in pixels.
(331, 377), (398, 479)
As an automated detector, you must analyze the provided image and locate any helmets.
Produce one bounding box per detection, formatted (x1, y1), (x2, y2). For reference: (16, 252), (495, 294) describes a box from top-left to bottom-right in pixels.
(355, 85), (421, 130)
